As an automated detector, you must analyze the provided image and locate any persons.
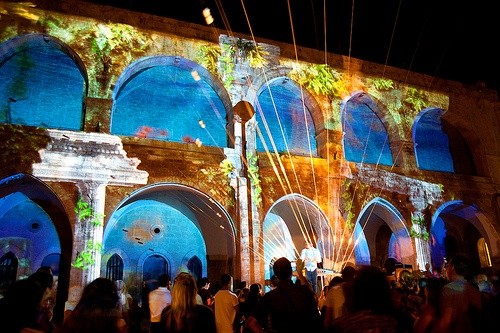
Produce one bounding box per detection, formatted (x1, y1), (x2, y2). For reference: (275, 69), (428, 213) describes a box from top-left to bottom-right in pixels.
(214, 275), (240, 333)
(160, 272), (216, 333)
(299, 240), (321, 295)
(264, 257), (326, 333)
(318, 256), (500, 333)
(0, 267), (134, 333)
(148, 274), (171, 333)
(197, 277), (215, 309)
(233, 279), (265, 333)
